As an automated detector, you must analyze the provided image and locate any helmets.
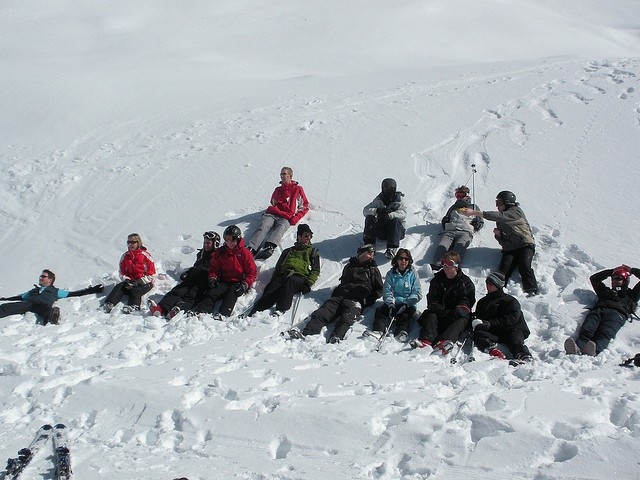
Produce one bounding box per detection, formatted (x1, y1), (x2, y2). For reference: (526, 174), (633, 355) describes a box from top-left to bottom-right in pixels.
(203, 231), (221, 249)
(611, 267), (628, 291)
(495, 191), (516, 212)
(223, 225), (241, 239)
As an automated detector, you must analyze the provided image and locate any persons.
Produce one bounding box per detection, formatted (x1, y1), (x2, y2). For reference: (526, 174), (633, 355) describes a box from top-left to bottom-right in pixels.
(237, 224), (321, 319)
(564, 264), (640, 357)
(186, 225), (257, 321)
(363, 248), (423, 343)
(362, 178), (408, 260)
(430, 185), (484, 271)
(147, 231), (221, 320)
(1, 269), (104, 325)
(464, 190), (538, 298)
(302, 243), (384, 344)
(409, 250), (476, 357)
(471, 270), (534, 367)
(103, 233), (156, 314)
(244, 167), (309, 259)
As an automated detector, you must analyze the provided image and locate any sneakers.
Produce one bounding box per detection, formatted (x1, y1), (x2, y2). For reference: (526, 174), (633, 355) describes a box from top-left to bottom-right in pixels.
(123, 304), (139, 315)
(288, 329), (305, 340)
(147, 300), (162, 316)
(509, 356), (533, 367)
(410, 339), (432, 349)
(384, 247), (397, 259)
(96, 302), (114, 314)
(167, 306), (180, 319)
(488, 348), (507, 359)
(432, 340), (450, 356)
(254, 242), (278, 260)
(564, 337), (582, 355)
(49, 307), (60, 324)
(394, 330), (409, 343)
(363, 331), (383, 341)
(328, 336), (340, 344)
(582, 341), (597, 356)
(245, 246), (257, 256)
(273, 309), (286, 317)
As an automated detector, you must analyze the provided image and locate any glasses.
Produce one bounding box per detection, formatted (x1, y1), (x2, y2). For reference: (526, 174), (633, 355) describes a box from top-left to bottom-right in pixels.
(283, 173), (286, 175)
(440, 259), (458, 268)
(127, 241), (137, 244)
(398, 256), (409, 261)
(40, 275), (47, 278)
(455, 192), (469, 198)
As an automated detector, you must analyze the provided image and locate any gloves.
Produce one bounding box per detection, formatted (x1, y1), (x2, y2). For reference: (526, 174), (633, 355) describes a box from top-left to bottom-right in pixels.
(125, 279), (133, 290)
(132, 279), (143, 287)
(389, 306), (396, 321)
(395, 303), (408, 316)
(88, 284), (104, 293)
(235, 283), (246, 297)
(299, 284), (310, 295)
(208, 279), (220, 288)
(180, 272), (189, 280)
(476, 320), (491, 331)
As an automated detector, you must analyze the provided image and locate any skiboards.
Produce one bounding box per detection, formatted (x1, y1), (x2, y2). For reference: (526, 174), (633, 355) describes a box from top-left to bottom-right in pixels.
(1, 423), (72, 480)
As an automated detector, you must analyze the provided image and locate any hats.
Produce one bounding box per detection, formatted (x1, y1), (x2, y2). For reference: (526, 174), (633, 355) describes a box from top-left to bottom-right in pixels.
(487, 271), (506, 290)
(297, 224), (313, 234)
(381, 178), (397, 202)
(357, 244), (376, 257)
